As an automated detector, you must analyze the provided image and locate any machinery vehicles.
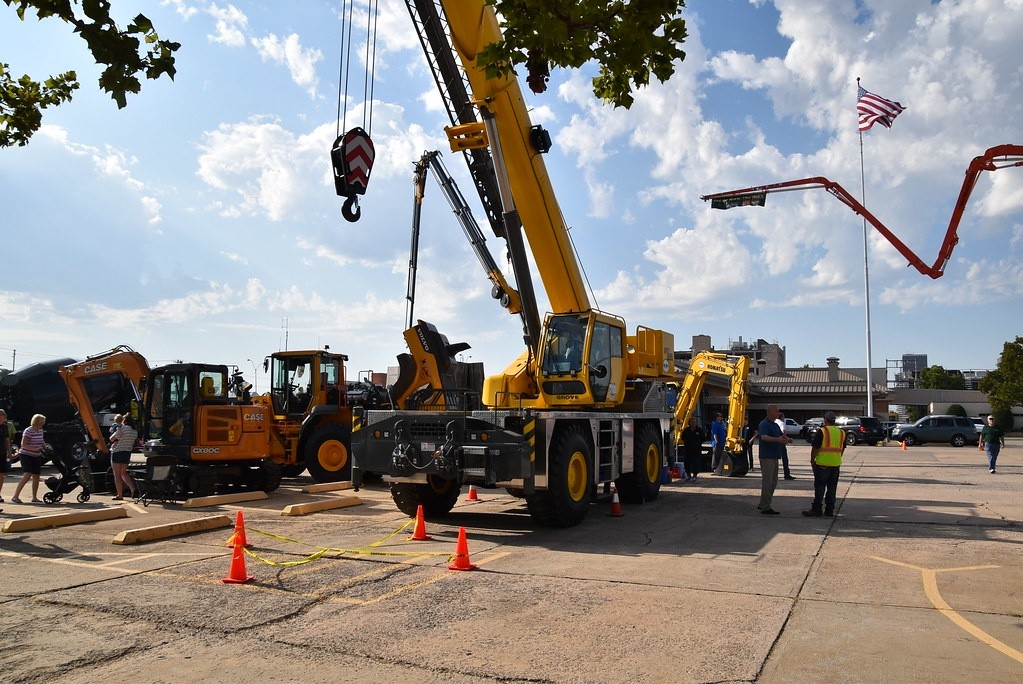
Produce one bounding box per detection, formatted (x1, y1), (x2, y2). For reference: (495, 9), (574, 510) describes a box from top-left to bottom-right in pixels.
(59, 344), (270, 494)
(349, 149), (522, 435)
(330, 0), (678, 527)
(670, 349), (750, 477)
(700, 143), (1023, 281)
(247, 348), (352, 481)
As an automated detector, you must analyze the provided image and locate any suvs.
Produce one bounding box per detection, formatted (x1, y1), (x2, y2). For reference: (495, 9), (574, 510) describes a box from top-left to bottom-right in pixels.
(892, 414), (979, 448)
(807, 416), (886, 446)
(970, 417), (986, 433)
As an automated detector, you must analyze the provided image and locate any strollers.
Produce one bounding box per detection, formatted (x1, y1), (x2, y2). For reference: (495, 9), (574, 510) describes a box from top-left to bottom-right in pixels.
(43, 446), (91, 505)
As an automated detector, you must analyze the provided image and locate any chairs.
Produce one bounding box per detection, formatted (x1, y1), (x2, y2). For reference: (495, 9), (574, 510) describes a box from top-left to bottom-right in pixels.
(134, 455), (179, 508)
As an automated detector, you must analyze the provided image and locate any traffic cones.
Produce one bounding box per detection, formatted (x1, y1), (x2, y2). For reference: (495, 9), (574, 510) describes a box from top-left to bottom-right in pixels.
(606, 488), (626, 518)
(447, 527), (478, 571)
(219, 537), (255, 584)
(900, 440), (909, 451)
(228, 511), (251, 549)
(464, 482), (482, 502)
(407, 505), (433, 540)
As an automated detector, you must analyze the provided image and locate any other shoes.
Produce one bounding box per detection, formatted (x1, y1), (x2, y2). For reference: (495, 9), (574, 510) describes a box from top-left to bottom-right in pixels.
(132, 489), (137, 499)
(990, 469), (996, 474)
(784, 475), (796, 480)
(758, 504), (780, 516)
(11, 496), (23, 503)
(112, 495), (123, 500)
(0, 496), (4, 502)
(685, 476), (698, 483)
(31, 498), (42, 502)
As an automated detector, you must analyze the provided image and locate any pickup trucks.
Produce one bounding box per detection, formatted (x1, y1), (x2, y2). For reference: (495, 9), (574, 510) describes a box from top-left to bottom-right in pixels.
(784, 419), (805, 439)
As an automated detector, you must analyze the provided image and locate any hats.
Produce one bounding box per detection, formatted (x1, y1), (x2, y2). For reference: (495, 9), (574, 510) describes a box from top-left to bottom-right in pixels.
(823, 412), (836, 420)
(988, 415), (995, 420)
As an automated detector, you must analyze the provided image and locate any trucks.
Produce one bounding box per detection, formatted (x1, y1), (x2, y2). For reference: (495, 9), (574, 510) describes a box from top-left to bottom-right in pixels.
(0, 356), (128, 472)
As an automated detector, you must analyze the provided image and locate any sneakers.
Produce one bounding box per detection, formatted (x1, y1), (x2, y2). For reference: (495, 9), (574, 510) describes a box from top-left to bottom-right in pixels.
(802, 508), (822, 516)
(824, 511), (833, 516)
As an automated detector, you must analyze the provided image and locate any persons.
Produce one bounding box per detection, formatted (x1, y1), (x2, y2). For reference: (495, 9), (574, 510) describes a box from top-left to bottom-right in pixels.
(758, 404), (794, 515)
(109, 412), (139, 500)
(775, 413), (796, 480)
(979, 415), (1004, 474)
(109, 414), (123, 453)
(681, 417), (706, 483)
(711, 412), (726, 473)
(802, 411), (847, 517)
(0, 409), (16, 513)
(12, 413), (47, 503)
(741, 416), (758, 472)
